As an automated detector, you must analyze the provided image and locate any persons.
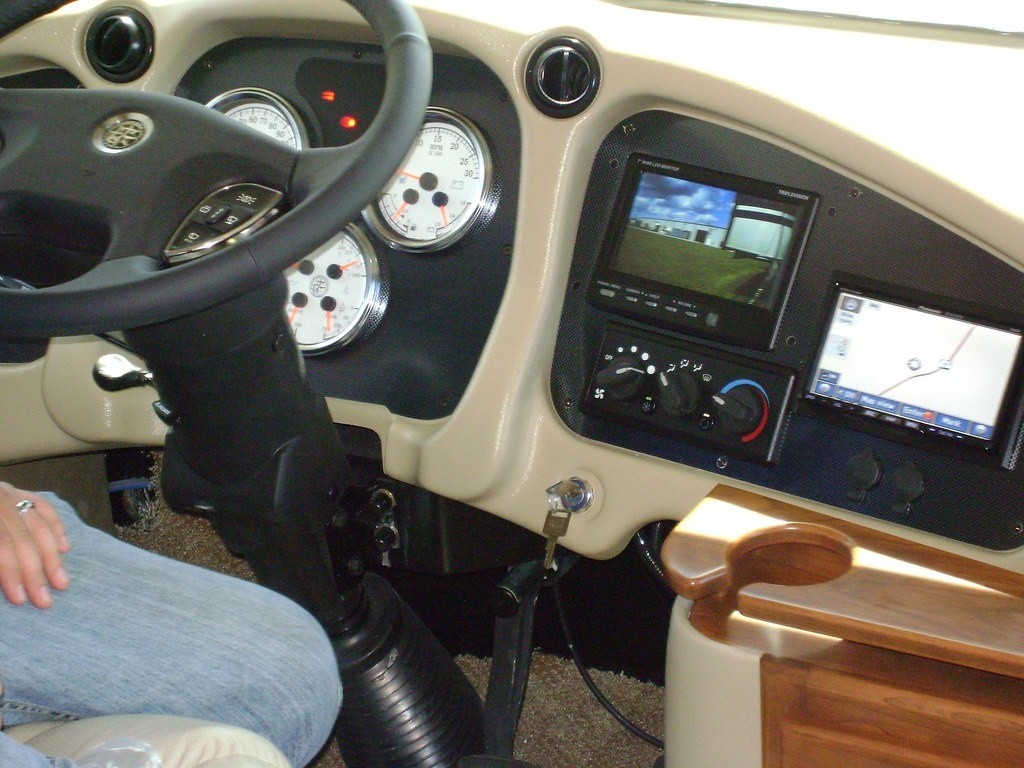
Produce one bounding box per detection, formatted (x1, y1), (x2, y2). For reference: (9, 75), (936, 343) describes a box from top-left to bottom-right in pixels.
(0, 481), (343, 768)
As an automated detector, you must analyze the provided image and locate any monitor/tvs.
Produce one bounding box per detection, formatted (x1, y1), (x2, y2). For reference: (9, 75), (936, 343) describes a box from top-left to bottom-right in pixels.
(592, 165), (808, 348)
(799, 285), (1024, 452)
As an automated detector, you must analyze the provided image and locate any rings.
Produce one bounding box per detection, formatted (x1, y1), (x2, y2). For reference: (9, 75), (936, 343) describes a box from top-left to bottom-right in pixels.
(14, 499), (35, 516)
